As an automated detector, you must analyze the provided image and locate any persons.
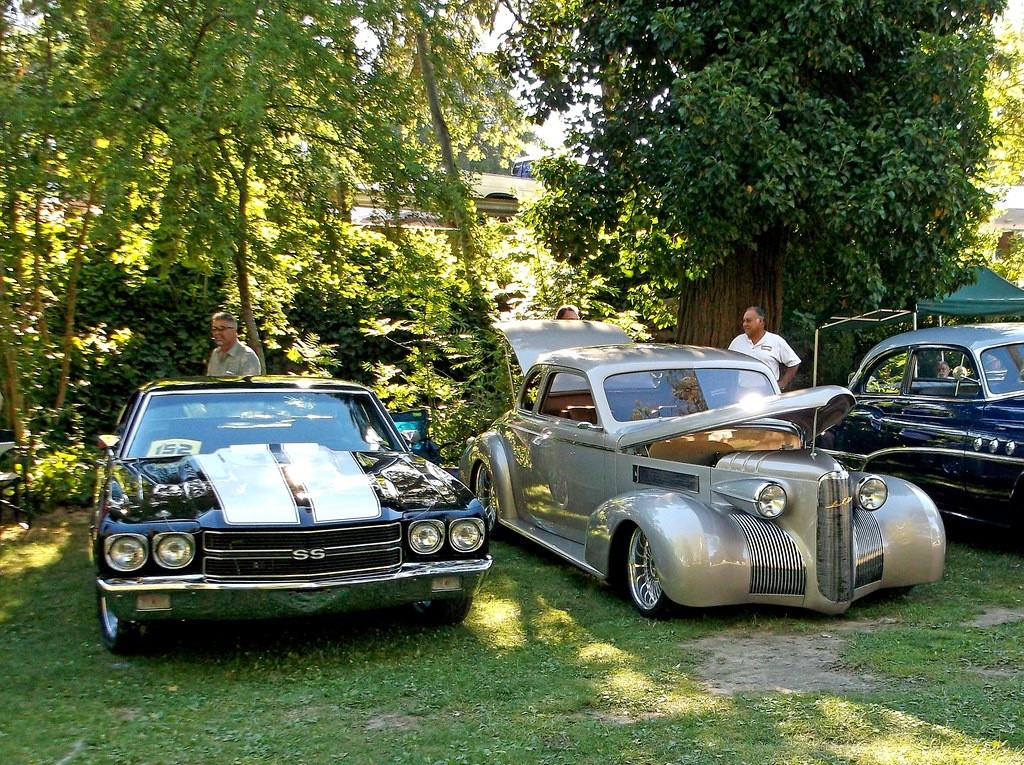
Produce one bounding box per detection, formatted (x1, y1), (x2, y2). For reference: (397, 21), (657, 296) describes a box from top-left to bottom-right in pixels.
(728, 307), (802, 401)
(556, 307), (578, 320)
(206, 312), (261, 416)
(848, 354), (1019, 395)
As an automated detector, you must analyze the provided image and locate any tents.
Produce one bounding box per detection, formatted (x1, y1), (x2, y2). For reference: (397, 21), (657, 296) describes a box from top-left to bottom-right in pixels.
(913, 270), (1024, 377)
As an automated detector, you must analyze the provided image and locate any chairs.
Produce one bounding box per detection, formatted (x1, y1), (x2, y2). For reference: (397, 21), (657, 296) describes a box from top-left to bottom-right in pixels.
(388, 410), (457, 474)
(147, 418), (344, 446)
(560, 403), (695, 423)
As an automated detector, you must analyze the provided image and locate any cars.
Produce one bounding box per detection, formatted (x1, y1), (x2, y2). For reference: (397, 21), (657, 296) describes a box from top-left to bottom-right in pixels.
(819, 322), (1024, 544)
(92, 376), (495, 654)
(455, 317), (947, 620)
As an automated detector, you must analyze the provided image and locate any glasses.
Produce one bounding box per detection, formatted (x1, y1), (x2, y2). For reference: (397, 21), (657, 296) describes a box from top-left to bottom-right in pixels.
(210, 326), (235, 332)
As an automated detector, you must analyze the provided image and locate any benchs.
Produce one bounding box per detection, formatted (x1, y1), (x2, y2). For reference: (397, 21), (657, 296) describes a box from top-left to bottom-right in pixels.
(907, 382), (1006, 397)
(0, 427), (31, 527)
